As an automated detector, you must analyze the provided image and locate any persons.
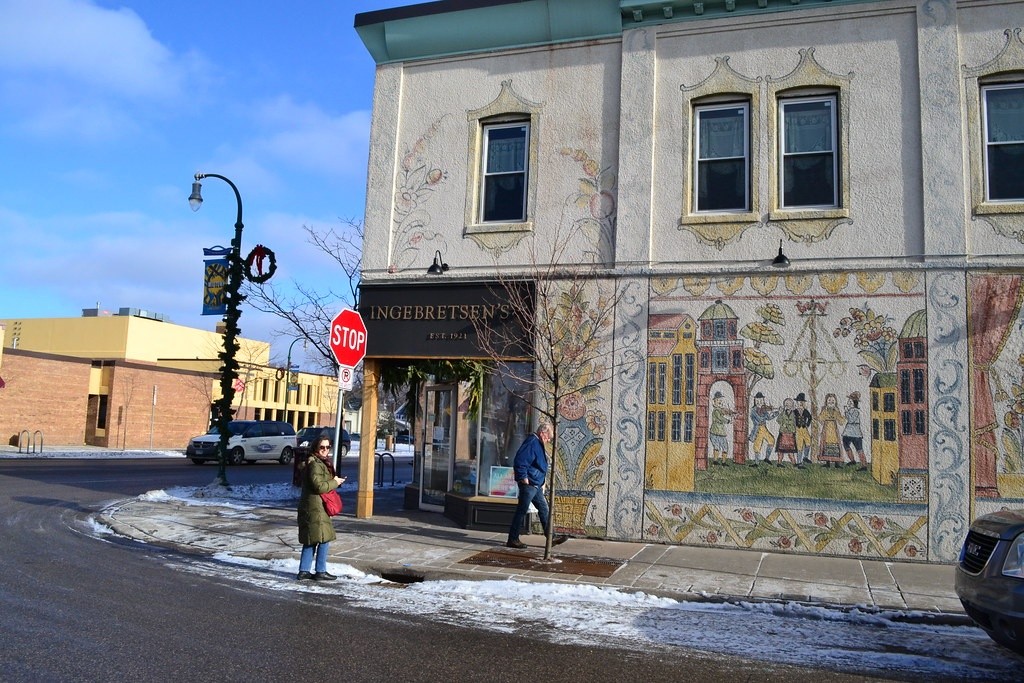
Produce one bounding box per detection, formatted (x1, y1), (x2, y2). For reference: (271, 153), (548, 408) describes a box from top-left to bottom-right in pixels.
(505, 423), (569, 548)
(297, 435), (347, 581)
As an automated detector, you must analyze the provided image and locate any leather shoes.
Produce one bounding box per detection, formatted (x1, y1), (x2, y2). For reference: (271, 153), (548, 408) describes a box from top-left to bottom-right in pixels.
(315, 572), (337, 580)
(297, 571), (315, 580)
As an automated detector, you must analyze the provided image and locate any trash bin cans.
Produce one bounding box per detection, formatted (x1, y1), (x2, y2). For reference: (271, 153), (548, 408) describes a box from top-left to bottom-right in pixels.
(385, 435), (393, 450)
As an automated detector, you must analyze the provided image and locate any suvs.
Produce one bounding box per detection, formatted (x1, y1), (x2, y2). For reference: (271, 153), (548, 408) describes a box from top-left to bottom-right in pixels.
(295, 425), (352, 457)
(185, 419), (298, 466)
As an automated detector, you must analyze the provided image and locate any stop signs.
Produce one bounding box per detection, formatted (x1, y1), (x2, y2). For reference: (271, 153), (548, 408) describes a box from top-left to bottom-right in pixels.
(328, 308), (367, 370)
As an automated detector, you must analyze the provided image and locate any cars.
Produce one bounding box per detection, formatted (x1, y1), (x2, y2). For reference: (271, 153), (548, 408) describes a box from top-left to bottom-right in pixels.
(954, 508), (1024, 655)
(392, 435), (414, 444)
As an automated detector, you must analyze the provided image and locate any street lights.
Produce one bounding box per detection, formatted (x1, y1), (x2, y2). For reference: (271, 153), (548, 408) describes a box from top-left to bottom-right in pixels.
(187, 171), (244, 493)
(282, 336), (309, 422)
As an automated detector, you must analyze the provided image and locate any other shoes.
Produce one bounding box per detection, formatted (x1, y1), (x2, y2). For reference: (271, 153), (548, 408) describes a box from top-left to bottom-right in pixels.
(552, 535), (569, 547)
(507, 540), (527, 549)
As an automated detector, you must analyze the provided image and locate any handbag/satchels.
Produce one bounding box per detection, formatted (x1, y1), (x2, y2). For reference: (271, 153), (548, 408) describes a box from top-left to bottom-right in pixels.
(321, 489), (343, 516)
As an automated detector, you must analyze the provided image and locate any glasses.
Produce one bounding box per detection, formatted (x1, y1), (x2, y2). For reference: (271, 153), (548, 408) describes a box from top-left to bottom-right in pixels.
(319, 445), (332, 450)
